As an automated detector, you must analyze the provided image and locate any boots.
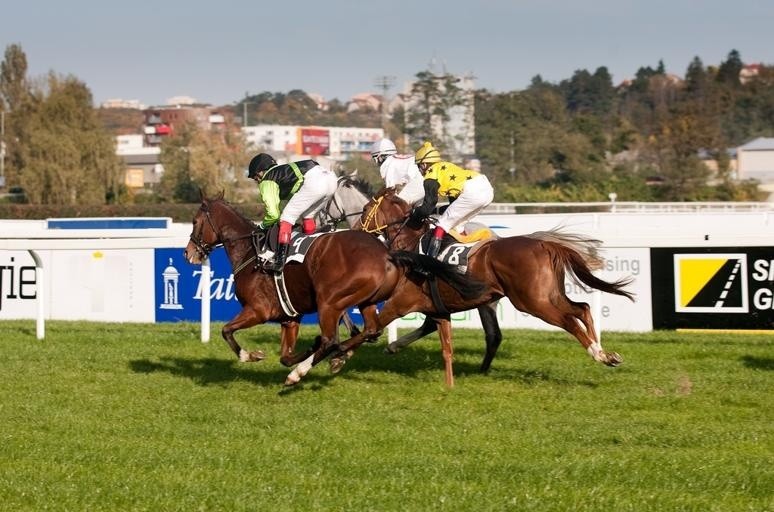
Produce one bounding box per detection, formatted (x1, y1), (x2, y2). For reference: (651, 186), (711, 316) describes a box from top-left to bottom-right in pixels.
(262, 242), (288, 274)
(427, 236), (443, 259)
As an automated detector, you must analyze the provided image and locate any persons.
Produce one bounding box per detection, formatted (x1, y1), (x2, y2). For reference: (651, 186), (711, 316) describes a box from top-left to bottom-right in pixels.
(248, 153), (338, 274)
(370, 138), (425, 243)
(404, 141), (495, 281)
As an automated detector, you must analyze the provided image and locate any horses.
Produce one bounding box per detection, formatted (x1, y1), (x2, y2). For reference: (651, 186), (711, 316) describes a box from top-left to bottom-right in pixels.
(184, 196), (485, 385)
(330, 184), (638, 383)
(312, 166), (605, 356)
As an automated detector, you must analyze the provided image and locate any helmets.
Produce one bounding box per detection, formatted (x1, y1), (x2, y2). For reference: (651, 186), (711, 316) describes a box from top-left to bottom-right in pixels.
(371, 138), (397, 156)
(415, 146), (441, 163)
(248, 153), (278, 178)
